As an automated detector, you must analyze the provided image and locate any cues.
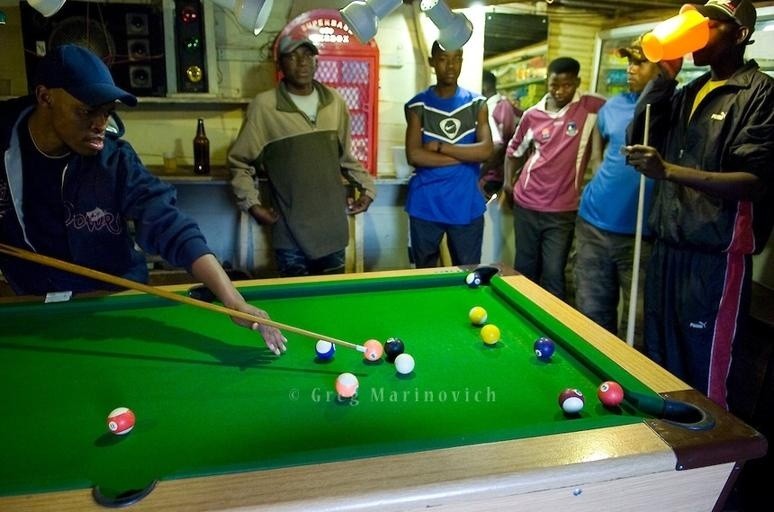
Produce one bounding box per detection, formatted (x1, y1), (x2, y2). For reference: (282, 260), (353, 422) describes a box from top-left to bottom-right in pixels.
(626, 103), (650, 347)
(0, 244), (368, 352)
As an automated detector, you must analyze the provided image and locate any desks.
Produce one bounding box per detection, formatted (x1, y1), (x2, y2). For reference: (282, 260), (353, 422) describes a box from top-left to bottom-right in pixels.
(1, 263), (771, 511)
(140, 164), (367, 275)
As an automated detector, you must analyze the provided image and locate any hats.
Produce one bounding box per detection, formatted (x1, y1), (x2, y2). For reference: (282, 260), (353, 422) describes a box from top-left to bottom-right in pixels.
(277, 35), (319, 57)
(616, 38), (648, 62)
(679, 0), (756, 44)
(33, 45), (137, 107)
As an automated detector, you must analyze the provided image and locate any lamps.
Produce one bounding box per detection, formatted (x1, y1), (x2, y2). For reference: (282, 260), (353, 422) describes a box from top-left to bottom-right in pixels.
(337, 1), (403, 46)
(209, 1), (274, 38)
(419, 1), (474, 54)
(23, 1), (64, 18)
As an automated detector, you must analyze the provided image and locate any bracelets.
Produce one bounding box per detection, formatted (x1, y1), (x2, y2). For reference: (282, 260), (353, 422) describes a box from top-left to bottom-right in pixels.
(436, 141), (442, 154)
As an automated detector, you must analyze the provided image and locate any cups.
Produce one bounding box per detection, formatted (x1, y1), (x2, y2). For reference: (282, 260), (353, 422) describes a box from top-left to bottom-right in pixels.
(391, 147), (412, 179)
(640, 10), (711, 64)
(163, 152), (177, 174)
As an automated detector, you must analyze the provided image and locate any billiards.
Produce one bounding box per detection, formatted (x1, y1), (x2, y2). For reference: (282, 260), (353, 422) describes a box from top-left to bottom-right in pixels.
(106, 407), (135, 435)
(315, 340), (336, 359)
(534, 337), (555, 358)
(394, 353), (415, 375)
(385, 338), (403, 357)
(559, 388), (584, 414)
(598, 381), (624, 406)
(469, 306), (487, 325)
(481, 324), (500, 345)
(466, 272), (481, 287)
(363, 339), (383, 361)
(335, 372), (360, 397)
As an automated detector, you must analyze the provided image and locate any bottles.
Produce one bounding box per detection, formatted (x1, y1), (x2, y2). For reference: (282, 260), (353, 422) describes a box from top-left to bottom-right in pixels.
(193, 118), (210, 175)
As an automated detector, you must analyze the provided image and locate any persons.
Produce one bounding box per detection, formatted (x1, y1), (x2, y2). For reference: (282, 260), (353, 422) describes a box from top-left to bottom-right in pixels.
(573, 29), (683, 357)
(0, 44), (287, 356)
(403, 41), (494, 268)
(625, 1), (773, 416)
(482, 69), (523, 204)
(226, 34), (377, 278)
(501, 57), (608, 302)
